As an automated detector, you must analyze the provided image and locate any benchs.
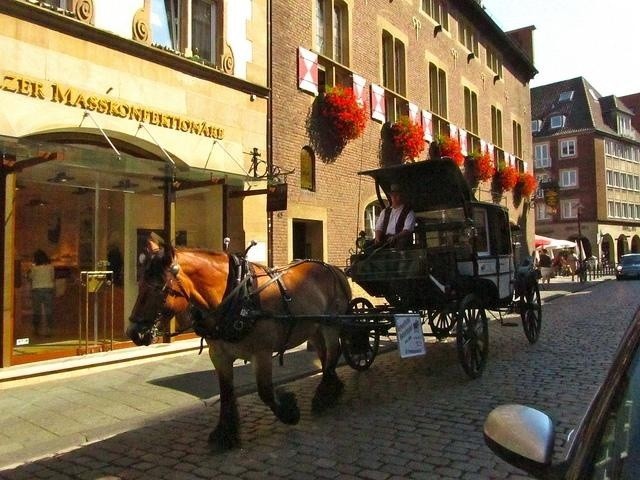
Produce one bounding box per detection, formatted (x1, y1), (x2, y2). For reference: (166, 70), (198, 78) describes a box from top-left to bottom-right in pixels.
(396, 222), (474, 280)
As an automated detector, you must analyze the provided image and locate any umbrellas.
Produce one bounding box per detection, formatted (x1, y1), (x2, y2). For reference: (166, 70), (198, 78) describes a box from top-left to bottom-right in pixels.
(536, 239), (577, 250)
(535, 235), (551, 250)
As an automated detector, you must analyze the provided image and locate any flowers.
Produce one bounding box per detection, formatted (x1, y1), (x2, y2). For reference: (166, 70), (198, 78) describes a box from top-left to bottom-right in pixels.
(317, 86), (538, 199)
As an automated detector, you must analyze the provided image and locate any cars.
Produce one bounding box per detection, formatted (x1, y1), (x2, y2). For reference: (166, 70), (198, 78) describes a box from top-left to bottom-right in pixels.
(614, 254), (640, 281)
(482, 303), (640, 480)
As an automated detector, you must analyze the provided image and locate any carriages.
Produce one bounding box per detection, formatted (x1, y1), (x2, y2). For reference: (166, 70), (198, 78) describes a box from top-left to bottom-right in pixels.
(126, 156), (543, 455)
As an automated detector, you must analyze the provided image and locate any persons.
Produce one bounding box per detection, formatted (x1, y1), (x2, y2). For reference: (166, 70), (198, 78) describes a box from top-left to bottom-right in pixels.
(589, 254), (599, 271)
(566, 250), (580, 274)
(540, 248), (554, 291)
(26, 248), (55, 339)
(555, 252), (564, 276)
(361, 184), (417, 253)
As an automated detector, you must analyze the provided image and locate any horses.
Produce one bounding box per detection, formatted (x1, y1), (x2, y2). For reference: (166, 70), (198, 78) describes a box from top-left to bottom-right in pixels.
(124, 236), (353, 452)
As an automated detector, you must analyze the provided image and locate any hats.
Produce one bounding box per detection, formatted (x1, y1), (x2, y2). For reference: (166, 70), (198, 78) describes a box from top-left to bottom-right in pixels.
(387, 183), (406, 196)
